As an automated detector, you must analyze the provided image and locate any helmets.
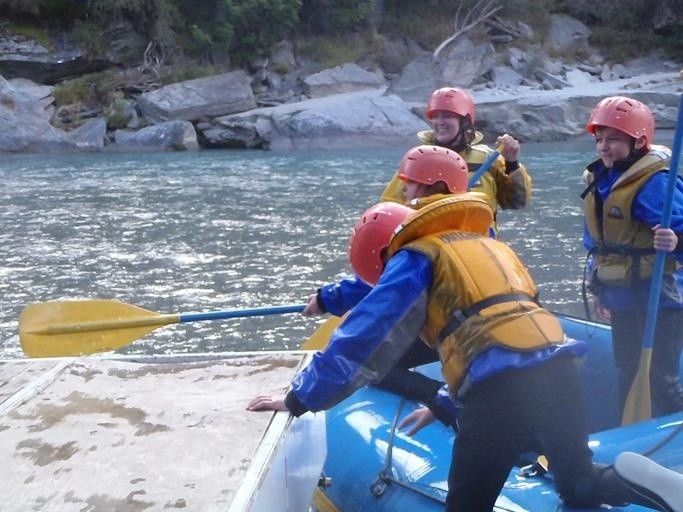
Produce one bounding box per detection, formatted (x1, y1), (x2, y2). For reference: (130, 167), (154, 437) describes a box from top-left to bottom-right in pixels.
(347, 201), (414, 288)
(396, 143), (470, 198)
(425, 86), (475, 124)
(586, 95), (656, 149)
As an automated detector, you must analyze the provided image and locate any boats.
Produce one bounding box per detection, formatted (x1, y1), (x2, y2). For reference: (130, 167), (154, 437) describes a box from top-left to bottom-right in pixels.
(297, 310), (681, 512)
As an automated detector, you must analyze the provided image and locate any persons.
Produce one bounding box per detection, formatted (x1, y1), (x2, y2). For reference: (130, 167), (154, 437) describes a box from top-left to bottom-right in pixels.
(580, 95), (683, 426)
(300, 142), (498, 404)
(245, 197), (683, 512)
(379, 85), (531, 226)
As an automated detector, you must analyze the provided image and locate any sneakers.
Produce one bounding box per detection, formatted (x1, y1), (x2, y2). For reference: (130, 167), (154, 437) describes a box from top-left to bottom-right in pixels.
(609, 449), (683, 512)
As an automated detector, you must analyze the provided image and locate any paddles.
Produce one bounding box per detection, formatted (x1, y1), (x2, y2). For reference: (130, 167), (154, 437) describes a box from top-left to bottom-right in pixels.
(623, 99), (682, 424)
(304, 146), (504, 351)
(19, 298), (308, 359)
